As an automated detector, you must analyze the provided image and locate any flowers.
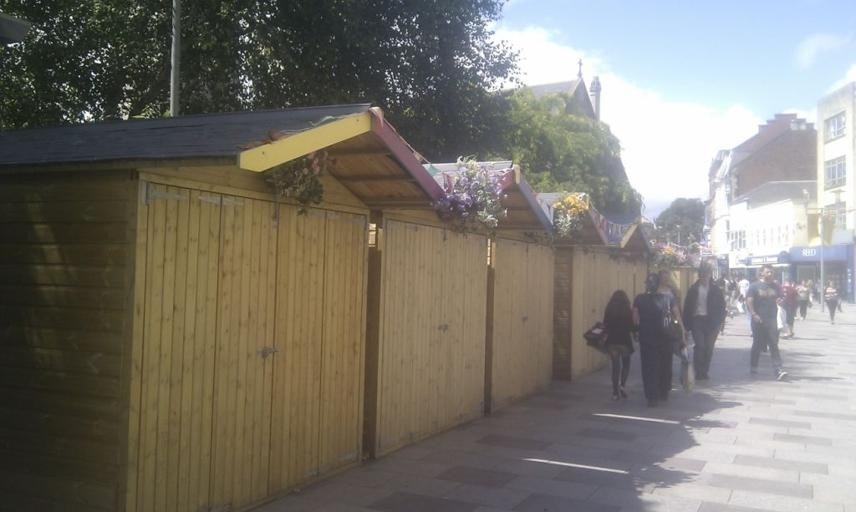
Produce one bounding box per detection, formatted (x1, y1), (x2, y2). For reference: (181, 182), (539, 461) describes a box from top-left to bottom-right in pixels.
(267, 150), (344, 217)
(653, 243), (680, 270)
(429, 154), (509, 237)
(529, 190), (589, 247)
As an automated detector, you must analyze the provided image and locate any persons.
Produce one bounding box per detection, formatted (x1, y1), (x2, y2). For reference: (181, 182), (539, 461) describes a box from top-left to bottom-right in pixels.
(601, 261), (843, 409)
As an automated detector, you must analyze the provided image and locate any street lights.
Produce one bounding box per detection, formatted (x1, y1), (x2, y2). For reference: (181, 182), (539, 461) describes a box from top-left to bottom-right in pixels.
(801, 188), (826, 312)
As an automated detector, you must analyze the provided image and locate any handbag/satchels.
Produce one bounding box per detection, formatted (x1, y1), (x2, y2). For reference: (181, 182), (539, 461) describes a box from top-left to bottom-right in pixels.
(679, 347), (695, 392)
(583, 322), (608, 350)
(662, 309), (682, 341)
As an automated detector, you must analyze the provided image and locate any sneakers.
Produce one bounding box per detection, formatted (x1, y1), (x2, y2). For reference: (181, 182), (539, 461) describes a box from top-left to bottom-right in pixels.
(618, 385), (626, 396)
(751, 367), (758, 376)
(648, 389), (667, 407)
(696, 372), (708, 379)
(776, 372), (786, 380)
(611, 395), (618, 400)
